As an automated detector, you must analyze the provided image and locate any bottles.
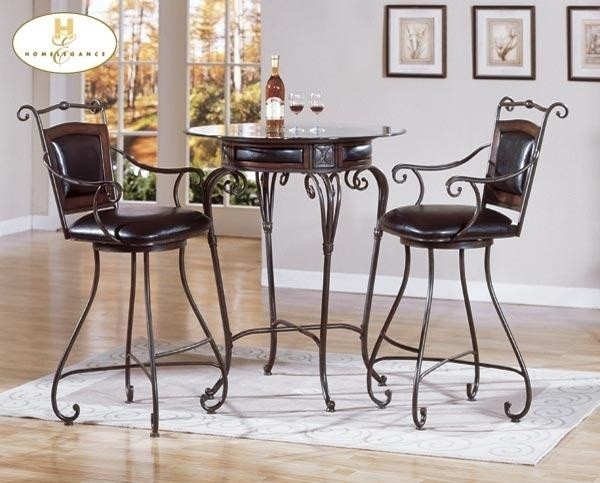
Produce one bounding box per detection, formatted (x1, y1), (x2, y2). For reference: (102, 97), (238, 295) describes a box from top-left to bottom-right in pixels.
(265, 54), (285, 139)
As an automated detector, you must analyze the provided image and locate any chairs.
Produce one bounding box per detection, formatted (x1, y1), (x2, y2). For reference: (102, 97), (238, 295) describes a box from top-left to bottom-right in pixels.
(17, 98), (568, 438)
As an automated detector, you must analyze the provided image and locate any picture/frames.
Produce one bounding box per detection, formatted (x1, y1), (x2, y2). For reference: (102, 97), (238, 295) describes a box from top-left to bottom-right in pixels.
(382, 6), (599, 82)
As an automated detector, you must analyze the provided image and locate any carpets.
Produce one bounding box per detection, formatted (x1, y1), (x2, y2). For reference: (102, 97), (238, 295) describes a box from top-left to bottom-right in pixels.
(2, 337), (598, 467)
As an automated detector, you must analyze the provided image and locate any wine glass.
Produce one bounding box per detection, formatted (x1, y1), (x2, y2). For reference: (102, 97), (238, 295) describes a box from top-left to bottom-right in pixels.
(289, 90), (327, 134)
